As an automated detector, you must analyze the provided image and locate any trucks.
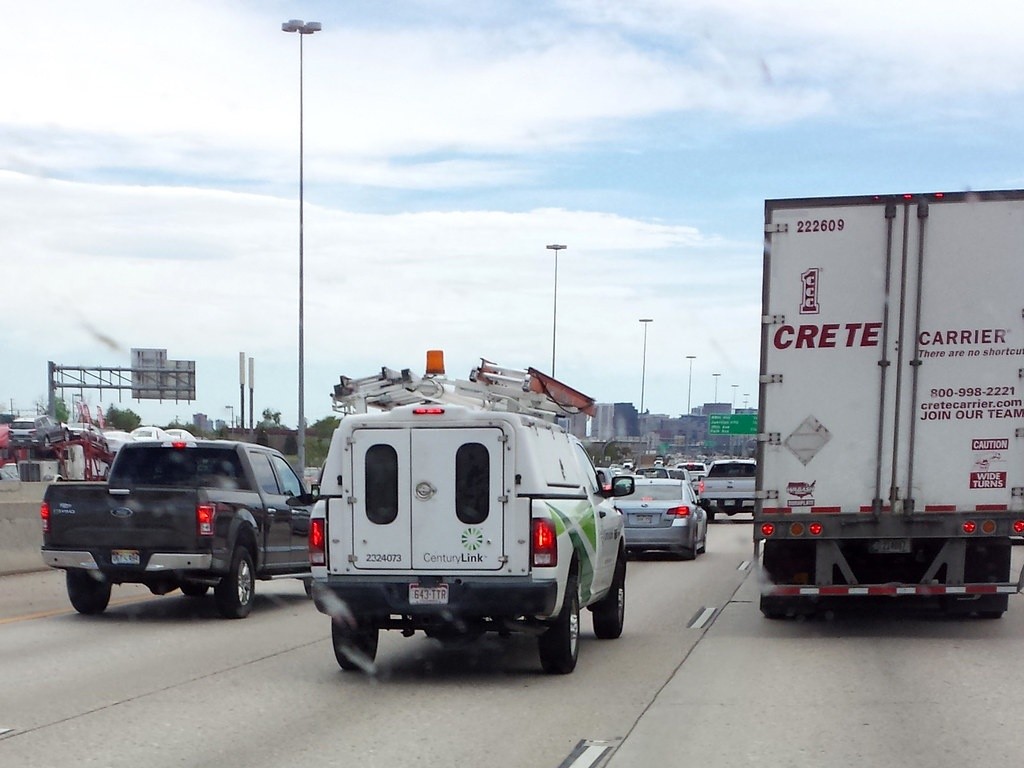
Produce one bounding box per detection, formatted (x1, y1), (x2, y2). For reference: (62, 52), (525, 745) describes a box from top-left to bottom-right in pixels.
(751, 188), (1024, 622)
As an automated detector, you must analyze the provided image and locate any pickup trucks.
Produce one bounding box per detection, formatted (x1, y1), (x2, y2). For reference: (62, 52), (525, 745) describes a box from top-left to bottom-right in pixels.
(697, 458), (757, 521)
(39, 439), (320, 619)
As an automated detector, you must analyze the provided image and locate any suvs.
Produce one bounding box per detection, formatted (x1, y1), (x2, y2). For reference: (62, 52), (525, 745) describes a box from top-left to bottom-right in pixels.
(308, 346), (637, 677)
(8, 414), (71, 451)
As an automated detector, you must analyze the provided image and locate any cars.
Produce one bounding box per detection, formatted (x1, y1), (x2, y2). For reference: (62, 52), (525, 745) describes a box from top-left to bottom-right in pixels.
(594, 454), (715, 500)
(613, 478), (711, 560)
(130, 426), (176, 443)
(66, 422), (110, 455)
(164, 428), (198, 442)
(54, 447), (111, 482)
(101, 429), (138, 454)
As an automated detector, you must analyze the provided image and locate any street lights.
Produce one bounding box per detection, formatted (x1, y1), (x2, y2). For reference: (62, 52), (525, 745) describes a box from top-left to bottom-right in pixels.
(684, 355), (697, 455)
(731, 384), (740, 414)
(637, 318), (654, 467)
(546, 244), (567, 378)
(280, 19), (324, 478)
(225, 406), (234, 440)
(712, 373), (721, 403)
